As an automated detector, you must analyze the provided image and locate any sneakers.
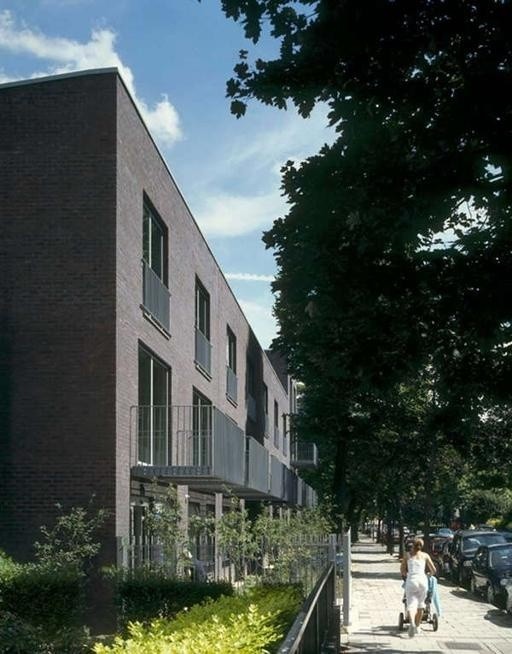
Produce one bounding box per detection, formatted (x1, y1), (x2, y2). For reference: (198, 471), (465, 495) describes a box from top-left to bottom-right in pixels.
(416, 628), (423, 636)
(408, 624), (416, 637)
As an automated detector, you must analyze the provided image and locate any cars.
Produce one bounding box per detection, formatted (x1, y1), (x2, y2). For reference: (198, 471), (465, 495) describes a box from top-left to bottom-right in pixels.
(365, 519), (512, 615)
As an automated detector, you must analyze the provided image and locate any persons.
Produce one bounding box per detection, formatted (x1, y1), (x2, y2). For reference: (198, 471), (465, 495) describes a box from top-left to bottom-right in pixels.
(401, 539), (437, 637)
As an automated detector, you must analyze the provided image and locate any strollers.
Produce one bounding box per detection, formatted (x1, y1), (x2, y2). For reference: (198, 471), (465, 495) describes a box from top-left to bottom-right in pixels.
(394, 573), (439, 630)
